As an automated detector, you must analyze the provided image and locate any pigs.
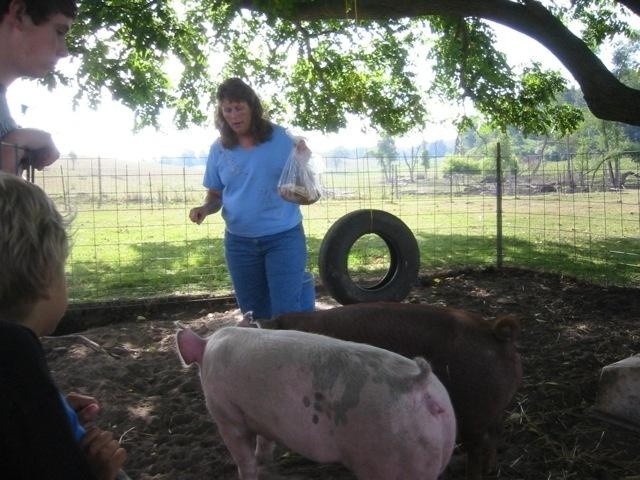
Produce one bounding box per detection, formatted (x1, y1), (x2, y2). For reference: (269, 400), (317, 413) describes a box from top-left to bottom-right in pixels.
(176, 312), (457, 480)
(256, 302), (523, 479)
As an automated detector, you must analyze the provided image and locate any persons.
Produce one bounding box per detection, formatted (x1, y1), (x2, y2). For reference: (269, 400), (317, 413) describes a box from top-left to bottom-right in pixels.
(1, 171), (129, 478)
(187, 75), (317, 324)
(0, 0), (80, 179)
